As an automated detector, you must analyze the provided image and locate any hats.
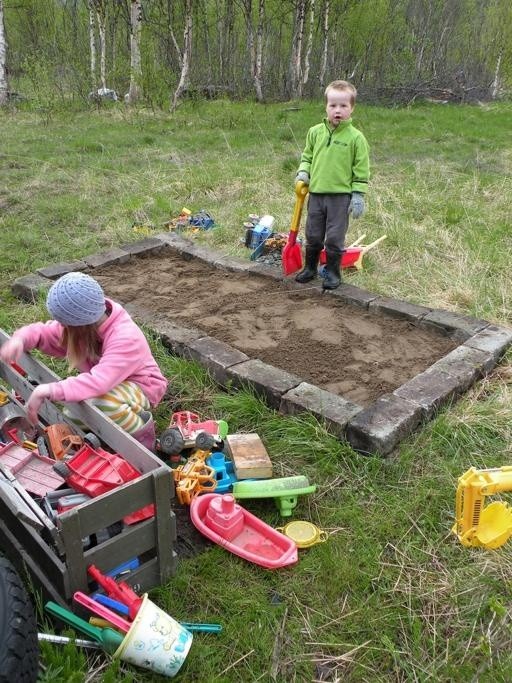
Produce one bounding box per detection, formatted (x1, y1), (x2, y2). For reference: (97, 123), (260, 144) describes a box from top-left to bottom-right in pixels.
(47, 273), (106, 327)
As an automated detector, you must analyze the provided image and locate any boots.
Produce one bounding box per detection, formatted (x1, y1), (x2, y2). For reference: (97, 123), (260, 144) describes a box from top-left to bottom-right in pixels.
(129, 412), (156, 453)
(296, 246), (321, 282)
(322, 252), (342, 289)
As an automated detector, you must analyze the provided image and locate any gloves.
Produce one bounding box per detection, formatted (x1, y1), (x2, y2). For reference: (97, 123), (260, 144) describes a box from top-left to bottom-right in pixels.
(294, 172), (310, 187)
(347, 193), (363, 219)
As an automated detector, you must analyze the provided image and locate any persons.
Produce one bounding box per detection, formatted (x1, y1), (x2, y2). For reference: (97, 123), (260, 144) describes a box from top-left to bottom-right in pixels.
(291, 79), (371, 290)
(0, 269), (169, 454)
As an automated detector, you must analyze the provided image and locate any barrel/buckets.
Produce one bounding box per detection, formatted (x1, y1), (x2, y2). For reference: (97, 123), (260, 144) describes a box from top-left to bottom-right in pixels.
(1, 402), (36, 444)
(110, 593), (195, 678)
